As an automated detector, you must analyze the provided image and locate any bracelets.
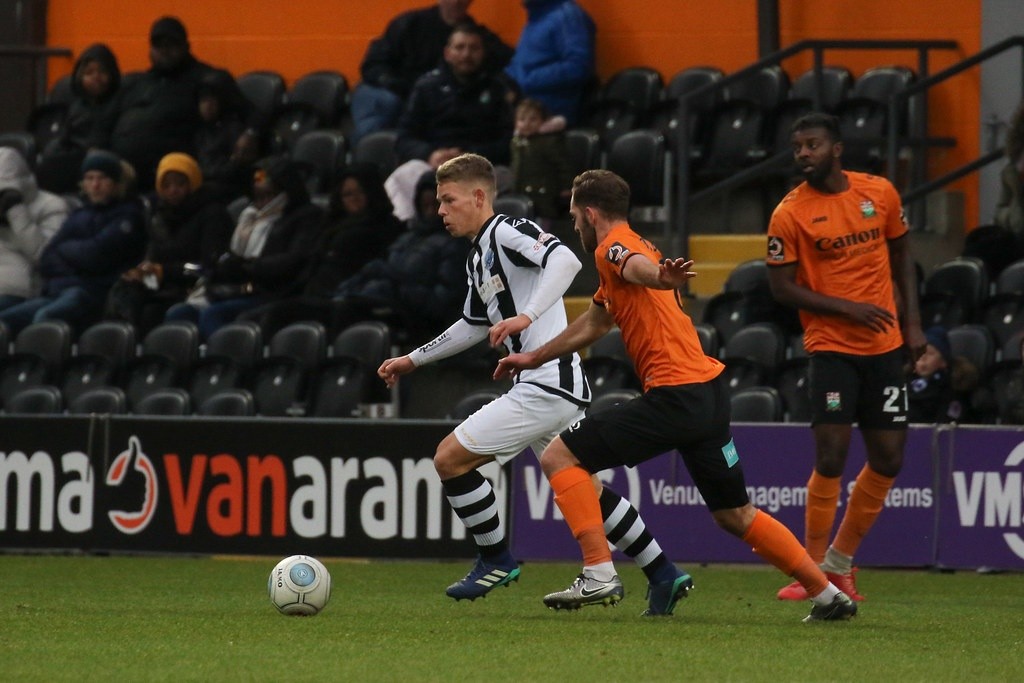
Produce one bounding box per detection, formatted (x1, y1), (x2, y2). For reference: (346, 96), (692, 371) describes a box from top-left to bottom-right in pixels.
(245, 127), (258, 136)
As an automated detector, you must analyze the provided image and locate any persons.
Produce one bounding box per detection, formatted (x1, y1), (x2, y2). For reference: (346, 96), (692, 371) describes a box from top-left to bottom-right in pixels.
(0, 146), (473, 350)
(493, 169), (857, 621)
(377, 155), (695, 616)
(359, 0), (596, 229)
(25, 17), (258, 193)
(907, 328), (973, 423)
(765, 111), (927, 602)
(992, 104), (1024, 234)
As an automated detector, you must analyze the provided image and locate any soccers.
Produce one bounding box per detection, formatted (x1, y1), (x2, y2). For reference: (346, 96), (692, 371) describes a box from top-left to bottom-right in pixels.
(266, 554), (334, 615)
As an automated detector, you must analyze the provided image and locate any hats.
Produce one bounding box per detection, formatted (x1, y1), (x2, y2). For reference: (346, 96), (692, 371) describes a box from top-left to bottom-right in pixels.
(83, 149), (125, 183)
(150, 16), (185, 38)
(924, 327), (951, 363)
(155, 152), (202, 200)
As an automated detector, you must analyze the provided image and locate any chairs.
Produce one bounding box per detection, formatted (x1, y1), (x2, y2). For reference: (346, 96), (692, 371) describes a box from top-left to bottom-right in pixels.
(0, 69), (1024, 420)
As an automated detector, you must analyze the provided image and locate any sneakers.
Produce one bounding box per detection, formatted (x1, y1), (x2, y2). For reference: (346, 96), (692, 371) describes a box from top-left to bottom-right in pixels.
(823, 566), (865, 601)
(801, 591), (858, 622)
(543, 573), (624, 613)
(446, 550), (521, 602)
(639, 563), (694, 617)
(778, 581), (812, 601)
